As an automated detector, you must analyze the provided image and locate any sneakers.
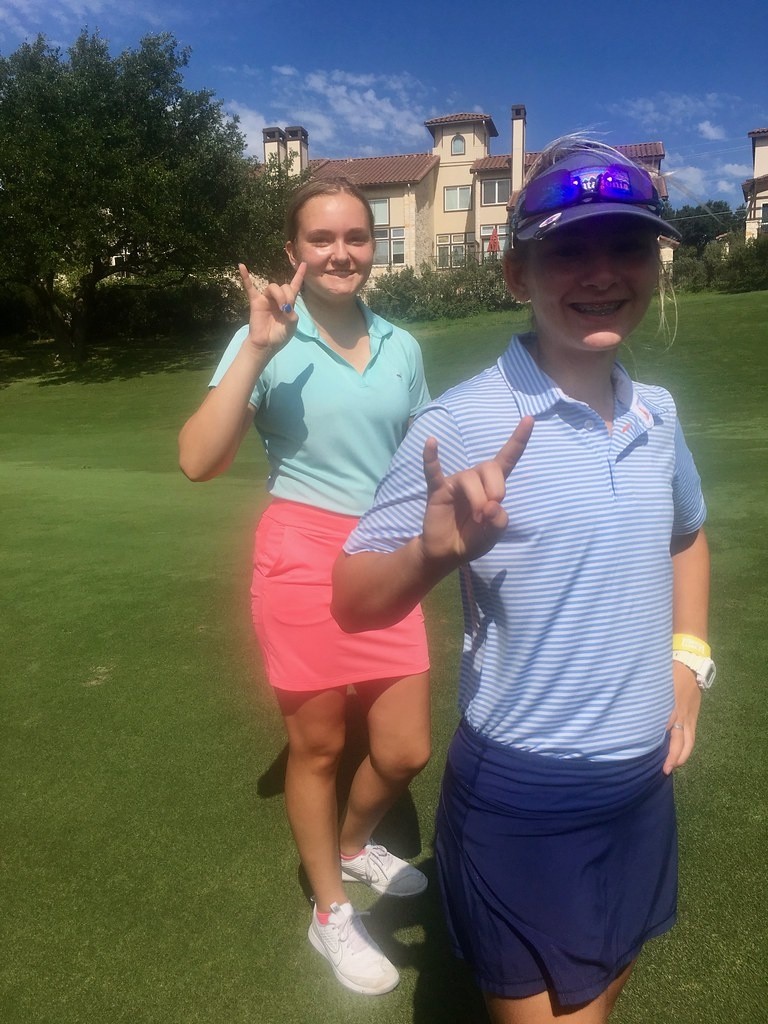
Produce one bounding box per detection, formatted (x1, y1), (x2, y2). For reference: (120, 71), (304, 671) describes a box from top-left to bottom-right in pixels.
(307, 894), (400, 995)
(338, 842), (429, 897)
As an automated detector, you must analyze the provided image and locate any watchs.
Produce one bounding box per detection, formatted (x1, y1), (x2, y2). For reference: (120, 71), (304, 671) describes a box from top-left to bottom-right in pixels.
(673, 648), (718, 690)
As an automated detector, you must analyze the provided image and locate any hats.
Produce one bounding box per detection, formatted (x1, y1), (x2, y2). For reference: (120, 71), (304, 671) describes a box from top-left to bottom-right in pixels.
(507, 147), (682, 241)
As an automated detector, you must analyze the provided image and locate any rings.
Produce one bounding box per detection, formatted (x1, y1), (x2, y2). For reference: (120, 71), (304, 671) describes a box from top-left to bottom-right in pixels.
(676, 724), (684, 730)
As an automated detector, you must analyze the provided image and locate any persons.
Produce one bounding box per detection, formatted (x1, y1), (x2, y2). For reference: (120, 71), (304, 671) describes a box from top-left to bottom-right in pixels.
(177, 173), (437, 995)
(330, 128), (713, 1024)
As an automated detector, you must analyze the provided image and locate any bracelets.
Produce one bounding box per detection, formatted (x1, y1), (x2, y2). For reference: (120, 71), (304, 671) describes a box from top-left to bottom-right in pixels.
(672, 632), (712, 658)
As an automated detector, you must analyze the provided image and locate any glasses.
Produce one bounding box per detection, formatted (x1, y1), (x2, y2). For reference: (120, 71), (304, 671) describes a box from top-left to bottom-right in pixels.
(510, 163), (665, 238)
